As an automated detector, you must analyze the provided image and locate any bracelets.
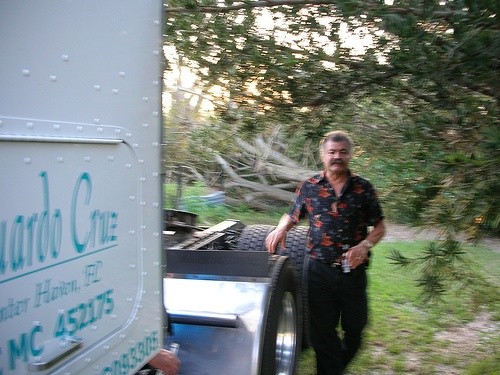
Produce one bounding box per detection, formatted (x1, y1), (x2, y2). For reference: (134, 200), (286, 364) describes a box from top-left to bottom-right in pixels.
(361, 240), (374, 249)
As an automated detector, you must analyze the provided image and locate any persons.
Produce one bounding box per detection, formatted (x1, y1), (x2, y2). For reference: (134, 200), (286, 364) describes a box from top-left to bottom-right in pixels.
(265, 131), (385, 375)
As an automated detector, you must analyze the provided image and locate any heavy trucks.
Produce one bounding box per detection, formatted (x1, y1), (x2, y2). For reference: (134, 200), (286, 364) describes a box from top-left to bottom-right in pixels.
(1, 1), (312, 375)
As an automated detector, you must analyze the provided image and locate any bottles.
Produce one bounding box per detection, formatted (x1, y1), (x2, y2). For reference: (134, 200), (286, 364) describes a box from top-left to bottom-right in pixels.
(341, 244), (352, 273)
